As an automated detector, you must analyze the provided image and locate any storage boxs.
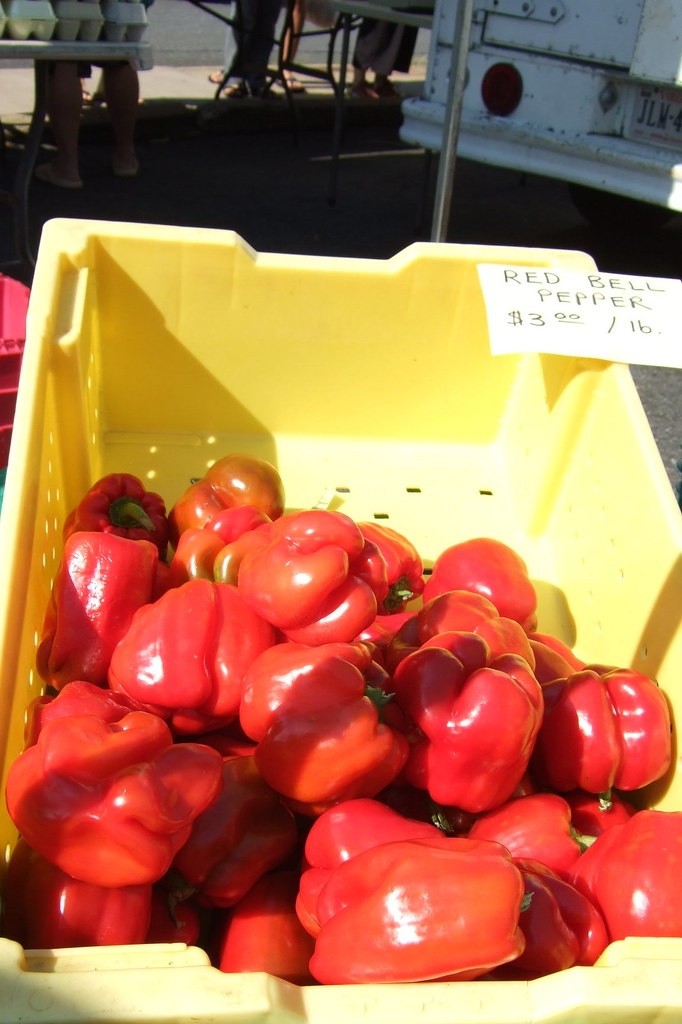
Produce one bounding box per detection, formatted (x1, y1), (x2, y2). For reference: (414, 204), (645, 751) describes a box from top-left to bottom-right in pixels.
(0, 271), (36, 469)
(0, 211), (682, 1024)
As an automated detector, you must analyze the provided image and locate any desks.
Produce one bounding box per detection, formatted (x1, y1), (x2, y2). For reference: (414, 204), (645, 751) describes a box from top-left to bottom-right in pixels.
(178, 0), (433, 208)
(0, 40), (166, 274)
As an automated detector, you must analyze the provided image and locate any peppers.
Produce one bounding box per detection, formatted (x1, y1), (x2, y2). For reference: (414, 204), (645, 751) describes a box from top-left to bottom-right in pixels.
(1, 450), (682, 987)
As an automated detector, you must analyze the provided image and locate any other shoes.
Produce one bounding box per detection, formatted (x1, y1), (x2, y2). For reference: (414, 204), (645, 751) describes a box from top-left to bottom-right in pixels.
(252, 87), (281, 100)
(348, 86), (374, 100)
(224, 86), (249, 98)
(373, 81), (400, 98)
(276, 71), (303, 91)
(209, 69), (226, 83)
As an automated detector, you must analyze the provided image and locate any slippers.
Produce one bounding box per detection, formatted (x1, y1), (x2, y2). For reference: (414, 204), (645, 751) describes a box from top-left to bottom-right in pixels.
(35, 162), (82, 189)
(113, 158), (139, 176)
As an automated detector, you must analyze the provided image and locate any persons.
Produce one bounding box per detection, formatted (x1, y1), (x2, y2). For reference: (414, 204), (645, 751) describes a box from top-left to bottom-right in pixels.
(36, 0), (154, 190)
(209, 0), (421, 100)
